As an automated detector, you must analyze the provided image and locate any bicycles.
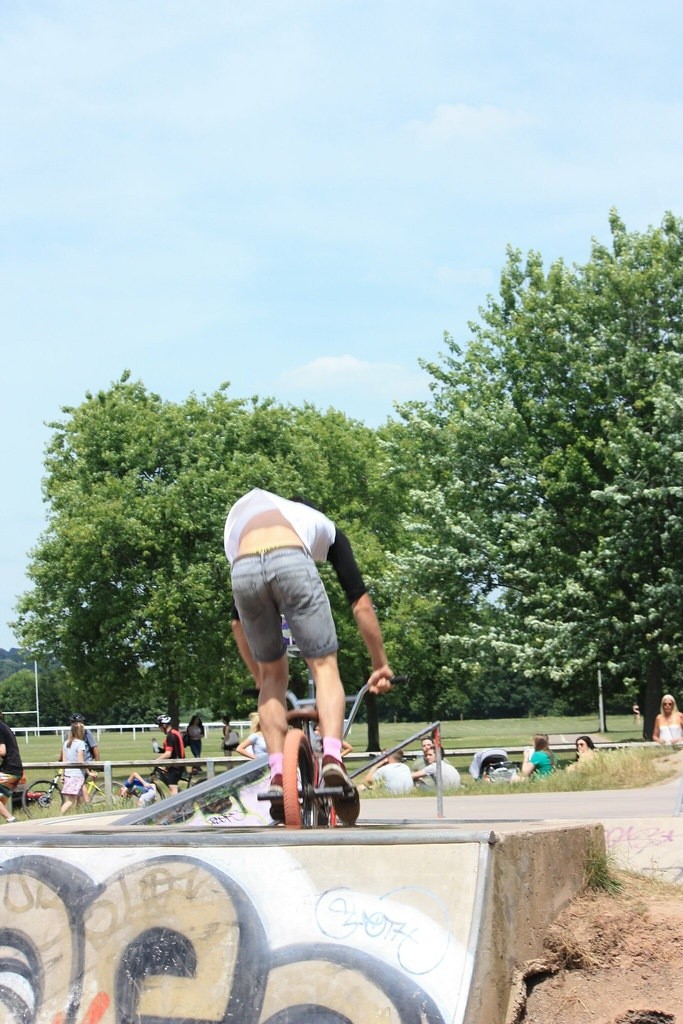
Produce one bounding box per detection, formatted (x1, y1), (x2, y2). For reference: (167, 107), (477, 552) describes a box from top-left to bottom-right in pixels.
(21, 760), (134, 820)
(238, 671), (410, 829)
(147, 763), (230, 821)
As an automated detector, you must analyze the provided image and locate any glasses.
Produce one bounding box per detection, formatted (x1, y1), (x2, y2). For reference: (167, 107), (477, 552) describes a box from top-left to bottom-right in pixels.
(576, 743), (587, 747)
(662, 702), (673, 706)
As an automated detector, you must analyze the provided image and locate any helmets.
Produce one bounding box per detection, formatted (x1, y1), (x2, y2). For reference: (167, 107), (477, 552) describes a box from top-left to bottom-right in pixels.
(156, 715), (172, 723)
(68, 713), (86, 721)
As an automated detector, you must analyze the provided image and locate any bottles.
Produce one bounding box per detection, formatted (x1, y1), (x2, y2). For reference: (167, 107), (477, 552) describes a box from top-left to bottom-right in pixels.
(152, 739), (159, 752)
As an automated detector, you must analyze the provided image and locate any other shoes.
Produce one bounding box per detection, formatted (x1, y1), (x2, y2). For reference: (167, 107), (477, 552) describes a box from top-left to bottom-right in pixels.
(269, 775), (286, 822)
(193, 767), (201, 773)
(3, 817), (17, 823)
(321, 754), (362, 821)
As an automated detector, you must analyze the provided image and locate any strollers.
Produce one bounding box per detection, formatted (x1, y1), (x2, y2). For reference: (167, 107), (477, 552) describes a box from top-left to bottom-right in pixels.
(468, 747), (522, 786)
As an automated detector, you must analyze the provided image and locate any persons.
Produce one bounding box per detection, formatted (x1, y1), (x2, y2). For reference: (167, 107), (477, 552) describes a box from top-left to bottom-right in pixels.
(68, 713), (100, 779)
(235, 712), (267, 760)
(313, 724), (353, 758)
(633, 702), (640, 724)
(522, 734), (557, 782)
(221, 715), (233, 771)
(61, 723), (98, 814)
(575, 736), (598, 763)
(187, 714), (205, 774)
(154, 715), (189, 824)
(0, 709), (24, 822)
(411, 736), (460, 793)
(120, 772), (159, 803)
(152, 738), (165, 753)
(652, 695), (683, 747)
(361, 746), (415, 795)
(224, 488), (395, 828)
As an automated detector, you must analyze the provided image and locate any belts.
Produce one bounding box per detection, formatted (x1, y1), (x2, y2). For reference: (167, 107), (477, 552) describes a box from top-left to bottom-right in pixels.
(234, 546), (303, 564)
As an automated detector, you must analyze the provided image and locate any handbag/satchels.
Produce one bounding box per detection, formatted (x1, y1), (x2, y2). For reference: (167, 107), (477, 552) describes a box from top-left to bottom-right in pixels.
(182, 734), (189, 747)
(225, 726), (239, 746)
